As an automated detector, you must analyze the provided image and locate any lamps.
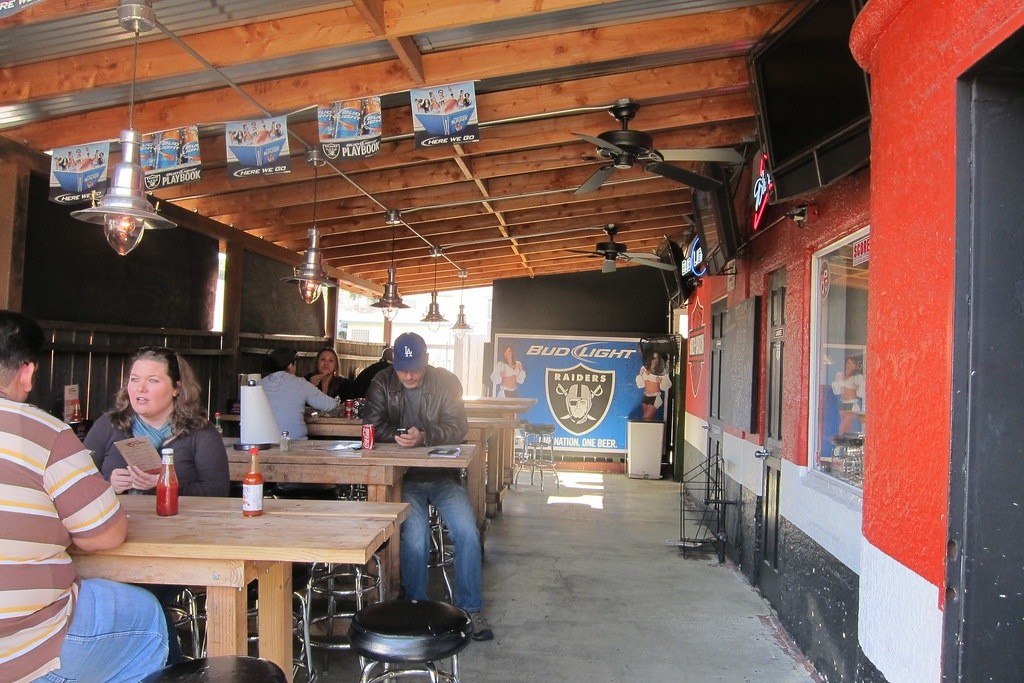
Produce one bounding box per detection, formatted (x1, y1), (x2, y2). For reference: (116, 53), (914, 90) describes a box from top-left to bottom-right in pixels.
(280, 146), (340, 307)
(372, 208), (409, 320)
(72, 6), (178, 255)
(451, 267), (474, 338)
(422, 244), (448, 330)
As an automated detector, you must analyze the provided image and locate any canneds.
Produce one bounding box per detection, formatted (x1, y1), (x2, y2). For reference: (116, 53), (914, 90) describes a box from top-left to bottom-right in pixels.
(345, 399), (354, 418)
(362, 424), (375, 449)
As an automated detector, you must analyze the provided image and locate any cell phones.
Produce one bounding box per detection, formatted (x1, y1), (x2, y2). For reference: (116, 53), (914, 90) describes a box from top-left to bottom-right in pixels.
(397, 429), (408, 436)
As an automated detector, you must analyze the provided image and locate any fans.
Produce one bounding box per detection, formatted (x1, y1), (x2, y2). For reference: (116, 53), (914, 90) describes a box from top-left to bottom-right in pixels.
(640, 336), (680, 374)
(570, 98), (747, 196)
(570, 224), (678, 274)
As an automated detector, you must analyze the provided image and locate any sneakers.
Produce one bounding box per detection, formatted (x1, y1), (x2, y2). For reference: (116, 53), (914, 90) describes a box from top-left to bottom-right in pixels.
(469, 611), (494, 642)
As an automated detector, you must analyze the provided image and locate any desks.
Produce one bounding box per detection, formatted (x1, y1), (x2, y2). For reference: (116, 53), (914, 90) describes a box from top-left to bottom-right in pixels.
(458, 396), (538, 418)
(211, 415), (509, 532)
(70, 492), (407, 683)
(224, 437), (476, 639)
(461, 417), (524, 489)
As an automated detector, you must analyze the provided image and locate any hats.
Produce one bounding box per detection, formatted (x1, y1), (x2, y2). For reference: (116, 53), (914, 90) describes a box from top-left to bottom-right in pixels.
(265, 347), (299, 372)
(393, 331), (427, 373)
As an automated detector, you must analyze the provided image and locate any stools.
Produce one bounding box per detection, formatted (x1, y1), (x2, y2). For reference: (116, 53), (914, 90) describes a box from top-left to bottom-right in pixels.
(133, 484), (474, 683)
(515, 426), (560, 493)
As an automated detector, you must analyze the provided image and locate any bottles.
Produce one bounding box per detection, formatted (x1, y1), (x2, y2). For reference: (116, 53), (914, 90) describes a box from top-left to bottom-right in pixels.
(71, 400), (83, 422)
(243, 448), (263, 517)
(279, 431), (291, 452)
(214, 413), (223, 436)
(157, 449), (179, 516)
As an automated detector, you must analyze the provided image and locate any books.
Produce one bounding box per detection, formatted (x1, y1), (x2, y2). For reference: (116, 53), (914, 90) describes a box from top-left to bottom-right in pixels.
(427, 448), (461, 458)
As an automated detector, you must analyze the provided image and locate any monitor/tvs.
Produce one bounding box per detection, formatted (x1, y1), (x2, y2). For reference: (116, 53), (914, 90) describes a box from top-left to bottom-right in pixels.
(743, 0), (874, 205)
(657, 241), (693, 310)
(690, 160), (743, 276)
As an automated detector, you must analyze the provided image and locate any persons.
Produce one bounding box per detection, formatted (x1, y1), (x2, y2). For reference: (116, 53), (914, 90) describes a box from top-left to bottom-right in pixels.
(832, 356), (864, 435)
(490, 343), (526, 398)
(354, 348), (394, 398)
(636, 352), (672, 420)
(304, 348), (356, 407)
(229, 119), (283, 145)
(0, 307), (169, 682)
(362, 332), (494, 641)
(256, 347), (336, 439)
(84, 346), (230, 666)
(54, 145), (104, 171)
(415, 85), (472, 113)
